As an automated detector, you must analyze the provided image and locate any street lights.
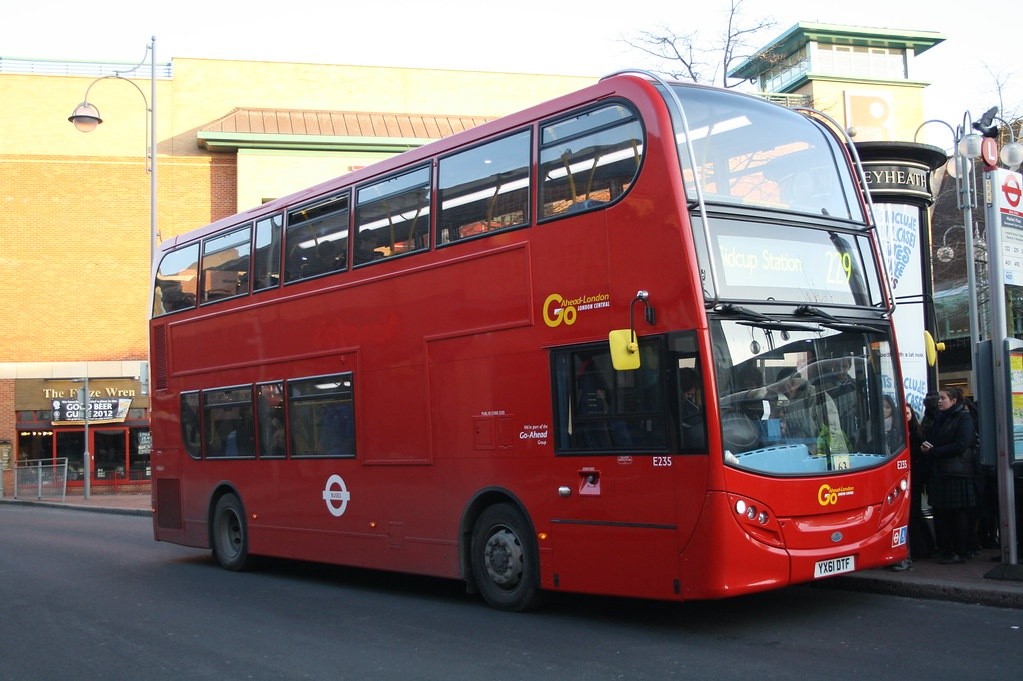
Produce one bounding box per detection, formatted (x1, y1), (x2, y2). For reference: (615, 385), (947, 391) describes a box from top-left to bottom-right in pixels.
(910, 108), (1023, 567)
(67, 76), (157, 295)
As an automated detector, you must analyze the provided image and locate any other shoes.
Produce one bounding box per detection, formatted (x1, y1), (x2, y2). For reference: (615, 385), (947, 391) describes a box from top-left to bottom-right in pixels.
(928, 550), (943, 560)
(966, 551), (975, 558)
(892, 560), (912, 572)
(939, 555), (961, 563)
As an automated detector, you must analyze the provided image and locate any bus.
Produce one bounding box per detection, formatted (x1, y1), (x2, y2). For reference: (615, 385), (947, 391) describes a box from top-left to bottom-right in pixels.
(149, 68), (945, 609)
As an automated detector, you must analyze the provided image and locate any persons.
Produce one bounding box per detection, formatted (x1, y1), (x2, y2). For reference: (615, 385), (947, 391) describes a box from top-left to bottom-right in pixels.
(644, 368), (703, 417)
(239, 228), (389, 291)
(822, 348), (859, 434)
(882, 386), (1000, 564)
(775, 368), (849, 454)
(185, 394), (356, 455)
(739, 366), (779, 420)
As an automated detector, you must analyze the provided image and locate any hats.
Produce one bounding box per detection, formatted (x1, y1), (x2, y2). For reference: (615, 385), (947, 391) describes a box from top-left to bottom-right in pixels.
(776, 368), (801, 382)
(738, 366), (762, 387)
(832, 349), (851, 370)
